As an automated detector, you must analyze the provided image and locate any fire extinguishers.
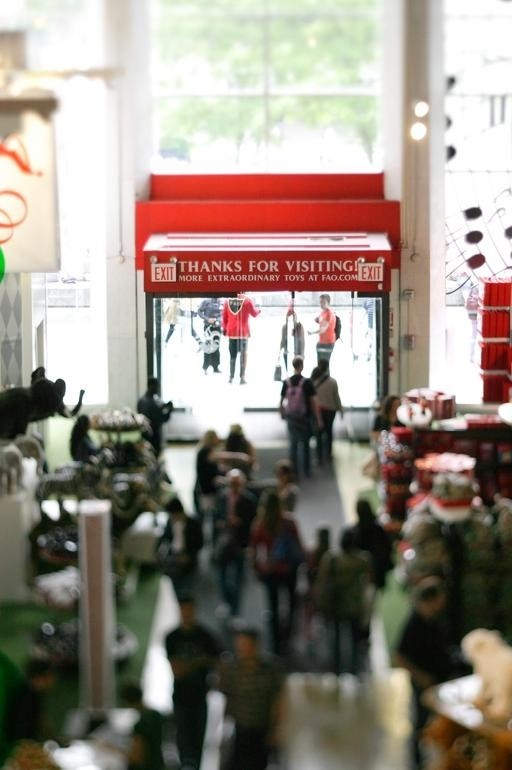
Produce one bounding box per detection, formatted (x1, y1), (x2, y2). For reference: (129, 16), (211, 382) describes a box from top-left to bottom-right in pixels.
(387, 347), (395, 372)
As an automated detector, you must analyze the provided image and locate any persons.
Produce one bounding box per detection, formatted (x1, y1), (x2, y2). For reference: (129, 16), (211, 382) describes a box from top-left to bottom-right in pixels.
(195, 298), (224, 376)
(363, 298), (373, 362)
(309, 358), (345, 465)
(221, 292), (264, 386)
(163, 296), (180, 349)
(0, 366), (510, 769)
(307, 292), (342, 374)
(279, 308), (304, 372)
(278, 356), (319, 479)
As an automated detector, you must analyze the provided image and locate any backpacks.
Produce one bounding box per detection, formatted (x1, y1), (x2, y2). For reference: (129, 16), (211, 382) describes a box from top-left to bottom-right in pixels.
(285, 378), (306, 416)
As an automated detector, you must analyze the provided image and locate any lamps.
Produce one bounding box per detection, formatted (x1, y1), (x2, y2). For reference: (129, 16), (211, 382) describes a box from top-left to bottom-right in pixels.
(409, 96), (432, 264)
